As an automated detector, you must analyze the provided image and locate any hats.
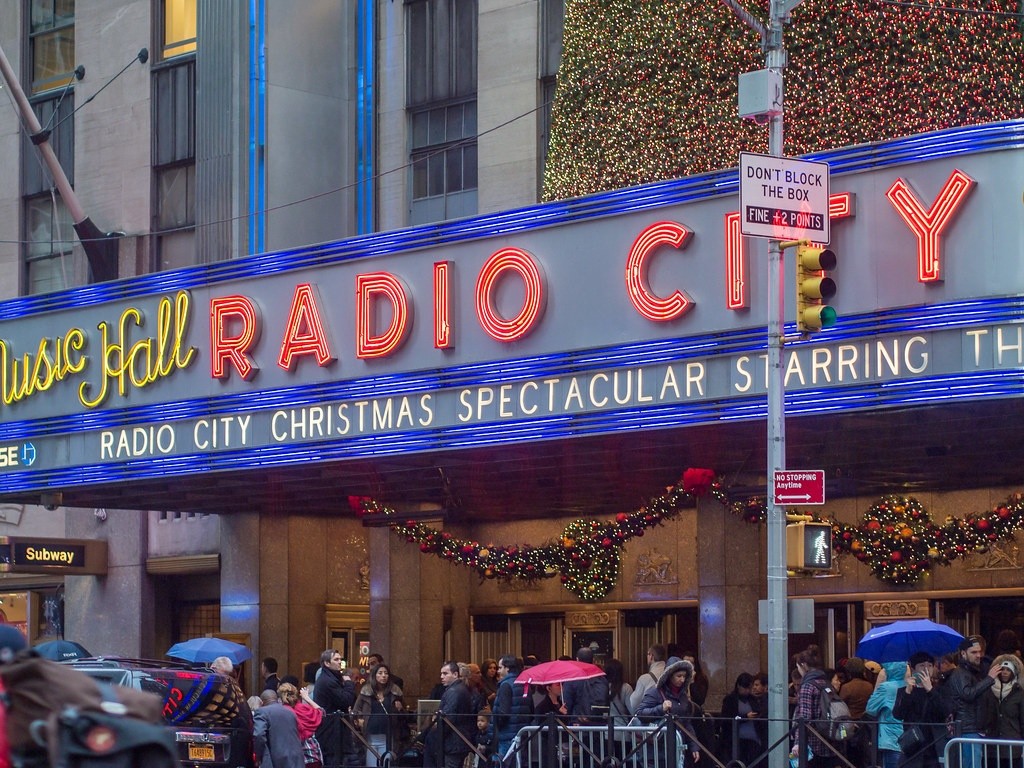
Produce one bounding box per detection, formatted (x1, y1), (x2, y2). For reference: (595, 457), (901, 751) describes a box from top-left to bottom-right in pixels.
(959, 636), (979, 651)
(1000, 661), (1015, 676)
(865, 661), (881, 674)
(845, 657), (863, 672)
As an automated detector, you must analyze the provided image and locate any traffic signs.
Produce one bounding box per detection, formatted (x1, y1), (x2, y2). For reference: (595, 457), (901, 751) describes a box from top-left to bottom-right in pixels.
(772, 469), (826, 507)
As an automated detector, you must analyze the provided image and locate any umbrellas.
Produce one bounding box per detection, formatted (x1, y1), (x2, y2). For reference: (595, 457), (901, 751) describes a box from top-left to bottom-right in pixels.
(513, 659), (607, 705)
(36, 640), (92, 662)
(166, 638), (253, 668)
(854, 620), (966, 677)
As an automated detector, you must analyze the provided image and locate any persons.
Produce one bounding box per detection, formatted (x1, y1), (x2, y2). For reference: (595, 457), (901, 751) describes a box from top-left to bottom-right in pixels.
(209, 629), (1024, 768)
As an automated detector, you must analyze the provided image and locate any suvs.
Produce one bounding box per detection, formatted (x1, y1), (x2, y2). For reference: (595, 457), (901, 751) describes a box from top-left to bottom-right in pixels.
(56, 656), (254, 768)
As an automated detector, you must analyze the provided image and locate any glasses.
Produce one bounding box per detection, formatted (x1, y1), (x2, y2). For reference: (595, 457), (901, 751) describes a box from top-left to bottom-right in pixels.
(333, 657), (345, 662)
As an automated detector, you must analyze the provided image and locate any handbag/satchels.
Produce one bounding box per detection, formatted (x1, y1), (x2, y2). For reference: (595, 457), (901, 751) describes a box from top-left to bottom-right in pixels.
(556, 741), (579, 761)
(627, 684), (668, 743)
(897, 726), (924, 754)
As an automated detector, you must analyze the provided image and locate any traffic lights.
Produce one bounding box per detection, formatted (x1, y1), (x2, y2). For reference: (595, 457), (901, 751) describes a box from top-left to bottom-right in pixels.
(784, 520), (834, 573)
(795, 245), (838, 334)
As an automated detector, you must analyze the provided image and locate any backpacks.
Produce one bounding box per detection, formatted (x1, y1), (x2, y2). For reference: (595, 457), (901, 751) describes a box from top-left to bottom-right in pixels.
(807, 679), (853, 741)
(582, 674), (611, 721)
